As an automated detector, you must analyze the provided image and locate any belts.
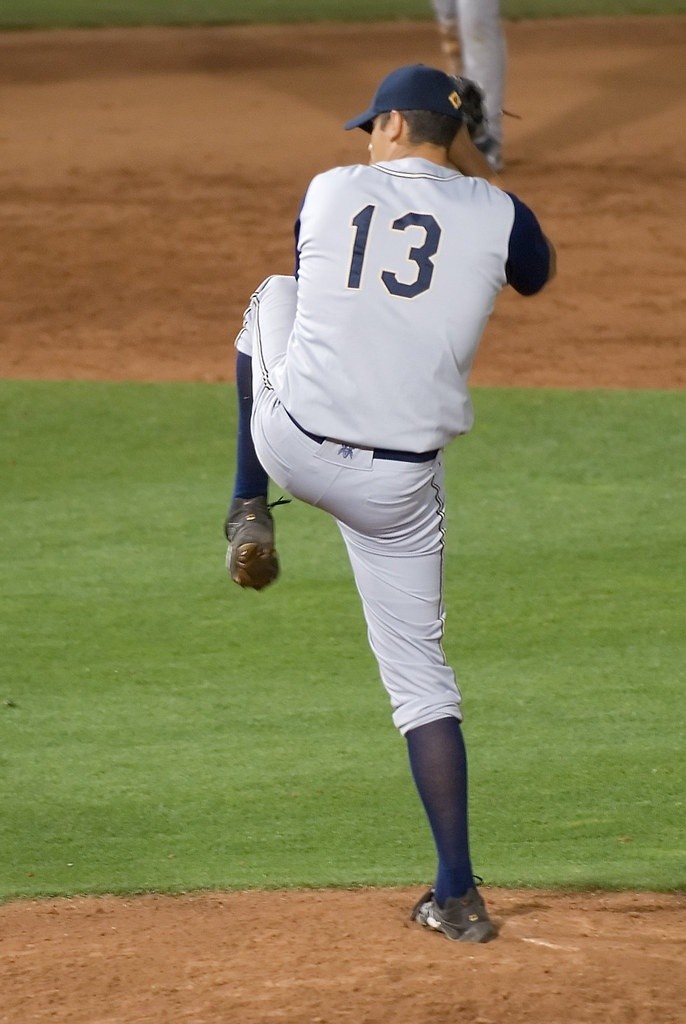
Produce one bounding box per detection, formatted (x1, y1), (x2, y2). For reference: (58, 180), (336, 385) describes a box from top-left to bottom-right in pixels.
(281, 402), (440, 463)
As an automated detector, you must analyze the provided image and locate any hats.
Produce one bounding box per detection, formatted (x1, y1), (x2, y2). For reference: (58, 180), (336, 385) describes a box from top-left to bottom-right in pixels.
(345, 64), (463, 135)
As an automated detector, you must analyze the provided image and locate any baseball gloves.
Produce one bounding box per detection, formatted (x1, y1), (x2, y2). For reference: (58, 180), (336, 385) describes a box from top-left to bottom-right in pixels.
(453, 73), (488, 144)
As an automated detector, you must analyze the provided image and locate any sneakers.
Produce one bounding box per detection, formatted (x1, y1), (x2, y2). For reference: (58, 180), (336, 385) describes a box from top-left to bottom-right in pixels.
(224, 494), (292, 594)
(409, 874), (498, 944)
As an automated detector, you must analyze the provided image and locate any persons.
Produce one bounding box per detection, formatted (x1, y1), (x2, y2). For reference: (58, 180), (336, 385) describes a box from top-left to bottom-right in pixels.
(431, 0), (505, 174)
(223, 62), (557, 945)
